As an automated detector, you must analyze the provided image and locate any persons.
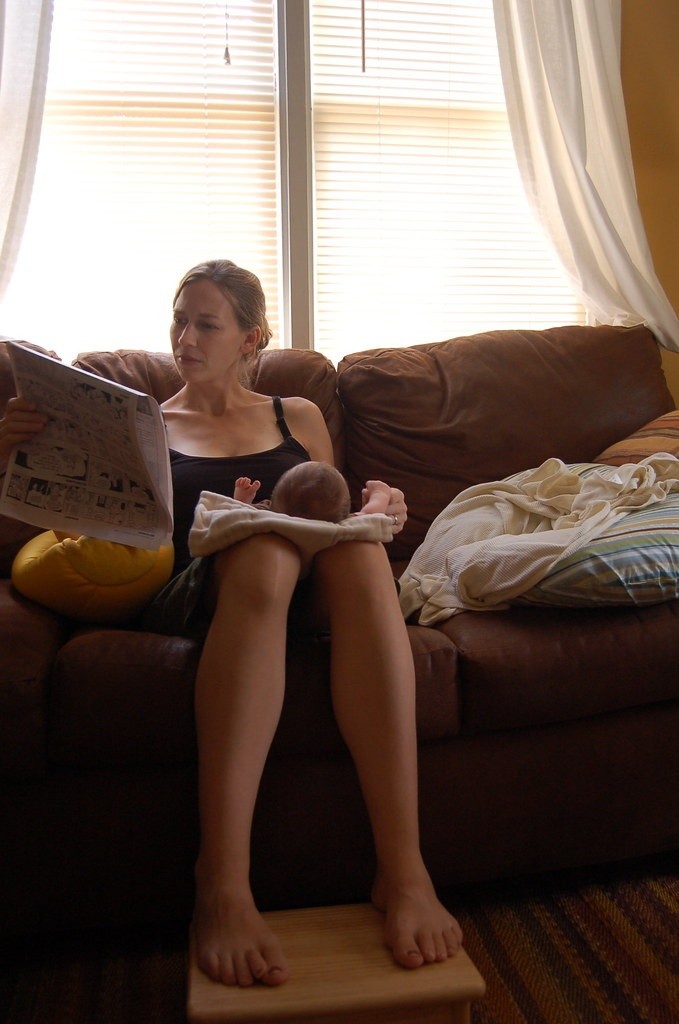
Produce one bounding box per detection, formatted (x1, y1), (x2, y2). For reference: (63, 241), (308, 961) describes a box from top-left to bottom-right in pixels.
(0, 260), (462, 985)
(233, 460), (391, 522)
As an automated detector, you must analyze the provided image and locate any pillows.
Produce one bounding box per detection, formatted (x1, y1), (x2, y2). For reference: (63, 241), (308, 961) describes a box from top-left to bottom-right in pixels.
(12, 531), (175, 621)
(446, 407), (679, 608)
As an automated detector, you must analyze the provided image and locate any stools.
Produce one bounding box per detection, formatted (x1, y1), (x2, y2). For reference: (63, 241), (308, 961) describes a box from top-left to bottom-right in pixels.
(187, 903), (486, 1024)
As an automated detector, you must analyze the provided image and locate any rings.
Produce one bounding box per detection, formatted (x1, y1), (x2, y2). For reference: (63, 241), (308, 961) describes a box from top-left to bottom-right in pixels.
(393, 514), (398, 525)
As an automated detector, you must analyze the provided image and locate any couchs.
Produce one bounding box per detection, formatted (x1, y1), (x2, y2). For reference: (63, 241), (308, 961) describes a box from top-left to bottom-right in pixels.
(0, 323), (679, 968)
